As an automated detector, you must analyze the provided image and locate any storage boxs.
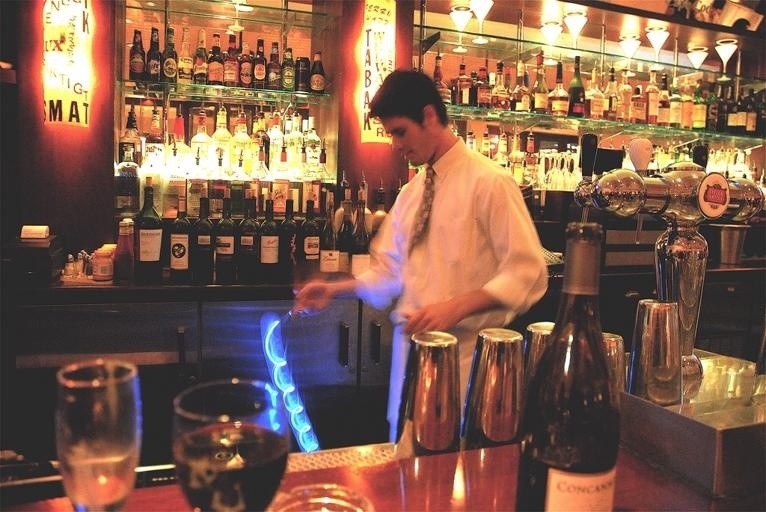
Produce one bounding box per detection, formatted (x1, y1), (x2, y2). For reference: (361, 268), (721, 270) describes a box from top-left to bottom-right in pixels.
(627, 338), (766, 503)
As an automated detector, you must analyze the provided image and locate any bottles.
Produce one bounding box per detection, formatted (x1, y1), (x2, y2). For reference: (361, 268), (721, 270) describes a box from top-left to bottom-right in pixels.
(117, 21), (329, 92)
(92, 176), (404, 288)
(433, 47), (766, 141)
(466, 131), (541, 189)
(513, 223), (621, 512)
(65, 251), (88, 281)
(114, 92), (329, 178)
(649, 145), (758, 186)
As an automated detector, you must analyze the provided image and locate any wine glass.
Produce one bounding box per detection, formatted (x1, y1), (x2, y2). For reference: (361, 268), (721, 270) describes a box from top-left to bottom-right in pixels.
(541, 147), (582, 191)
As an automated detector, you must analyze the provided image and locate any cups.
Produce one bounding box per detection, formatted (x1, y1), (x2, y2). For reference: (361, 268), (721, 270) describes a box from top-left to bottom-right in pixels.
(712, 221), (753, 267)
(522, 320), (556, 411)
(460, 328), (524, 446)
(628, 300), (685, 412)
(600, 334), (626, 413)
(461, 448), (524, 512)
(395, 330), (459, 455)
(54, 356), (145, 511)
(271, 482), (378, 511)
(170, 378), (289, 511)
(398, 454), (458, 511)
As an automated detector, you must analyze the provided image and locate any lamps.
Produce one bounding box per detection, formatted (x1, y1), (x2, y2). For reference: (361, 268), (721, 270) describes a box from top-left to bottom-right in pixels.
(38, 0), (92, 130)
(353, 0), (400, 150)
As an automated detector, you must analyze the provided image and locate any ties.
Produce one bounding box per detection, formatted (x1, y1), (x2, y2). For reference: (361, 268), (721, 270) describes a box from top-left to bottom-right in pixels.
(407, 168), (438, 257)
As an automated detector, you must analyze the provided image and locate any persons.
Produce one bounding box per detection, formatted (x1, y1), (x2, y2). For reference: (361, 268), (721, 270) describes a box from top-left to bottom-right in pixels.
(288, 67), (550, 445)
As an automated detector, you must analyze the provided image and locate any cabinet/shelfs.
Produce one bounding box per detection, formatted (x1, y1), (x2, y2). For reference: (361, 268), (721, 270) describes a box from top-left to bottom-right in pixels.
(106, 1), (346, 231)
(507, 267), (566, 333)
(598, 267), (663, 354)
(695, 262), (766, 371)
(406, 0), (765, 221)
(0, 283), (197, 422)
(193, 280), (398, 418)
